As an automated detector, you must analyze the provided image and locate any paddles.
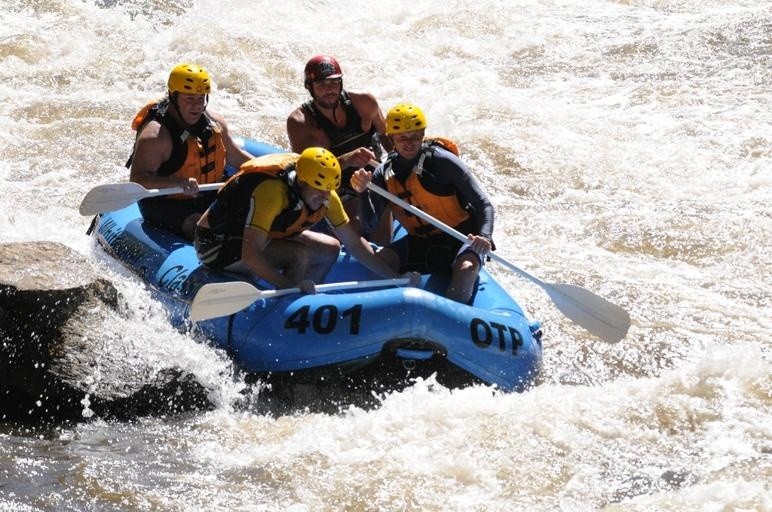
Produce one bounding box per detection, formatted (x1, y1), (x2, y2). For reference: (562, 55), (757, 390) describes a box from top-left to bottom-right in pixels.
(366, 182), (631, 345)
(79, 181), (226, 216)
(191, 274), (431, 321)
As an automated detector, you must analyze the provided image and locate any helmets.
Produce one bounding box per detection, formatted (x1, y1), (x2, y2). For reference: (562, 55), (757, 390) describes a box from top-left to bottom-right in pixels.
(297, 147), (341, 191)
(305, 56), (342, 81)
(387, 103), (426, 133)
(168, 65), (211, 95)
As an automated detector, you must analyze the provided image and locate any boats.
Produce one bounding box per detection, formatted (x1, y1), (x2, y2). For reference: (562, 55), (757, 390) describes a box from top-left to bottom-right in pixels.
(91, 133), (550, 399)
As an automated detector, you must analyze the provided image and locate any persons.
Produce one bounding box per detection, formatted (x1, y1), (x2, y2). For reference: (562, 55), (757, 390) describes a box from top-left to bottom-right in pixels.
(129, 62), (257, 246)
(350, 102), (495, 305)
(287, 54), (397, 247)
(193, 146), (422, 295)
